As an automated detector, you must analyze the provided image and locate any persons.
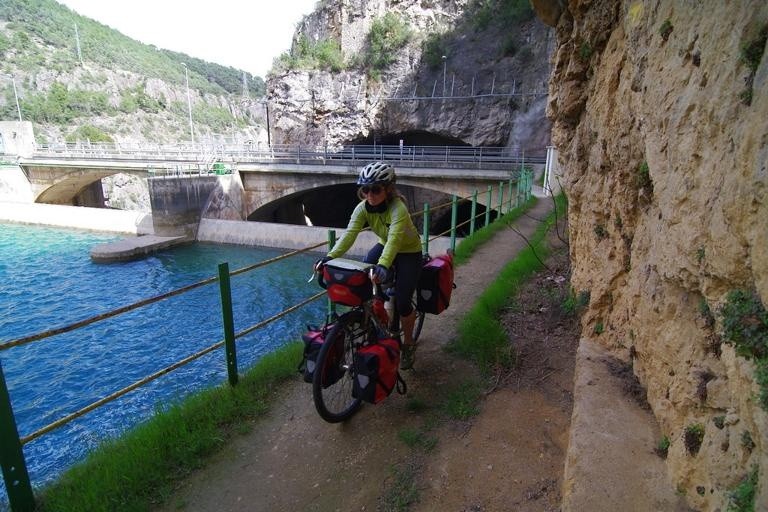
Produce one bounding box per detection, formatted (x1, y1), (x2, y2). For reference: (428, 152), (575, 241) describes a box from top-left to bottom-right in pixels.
(313, 161), (422, 370)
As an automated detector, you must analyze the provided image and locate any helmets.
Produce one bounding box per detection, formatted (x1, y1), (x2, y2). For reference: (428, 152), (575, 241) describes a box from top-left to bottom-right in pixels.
(356, 161), (397, 184)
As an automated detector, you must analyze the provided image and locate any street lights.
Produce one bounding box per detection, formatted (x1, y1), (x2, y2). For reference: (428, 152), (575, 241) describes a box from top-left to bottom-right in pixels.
(9, 72), (24, 122)
(261, 99), (270, 151)
(180, 60), (195, 154)
(441, 53), (447, 96)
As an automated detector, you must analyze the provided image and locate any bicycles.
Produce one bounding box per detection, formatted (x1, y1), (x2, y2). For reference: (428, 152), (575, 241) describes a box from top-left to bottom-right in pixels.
(297, 253), (427, 426)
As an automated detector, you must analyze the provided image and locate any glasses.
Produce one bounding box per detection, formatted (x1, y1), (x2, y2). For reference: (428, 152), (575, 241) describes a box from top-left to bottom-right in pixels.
(360, 185), (388, 195)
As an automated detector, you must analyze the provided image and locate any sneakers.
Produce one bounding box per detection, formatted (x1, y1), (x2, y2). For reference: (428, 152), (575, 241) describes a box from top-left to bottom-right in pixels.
(399, 339), (418, 370)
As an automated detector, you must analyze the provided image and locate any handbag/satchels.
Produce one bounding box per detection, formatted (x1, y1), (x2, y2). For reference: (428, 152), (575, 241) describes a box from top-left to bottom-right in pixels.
(351, 337), (408, 405)
(297, 317), (346, 388)
(416, 255), (456, 315)
(323, 257), (378, 307)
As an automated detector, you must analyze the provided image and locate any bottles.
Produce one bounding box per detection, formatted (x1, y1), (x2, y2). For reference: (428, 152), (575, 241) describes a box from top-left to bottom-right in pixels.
(373, 295), (395, 324)
